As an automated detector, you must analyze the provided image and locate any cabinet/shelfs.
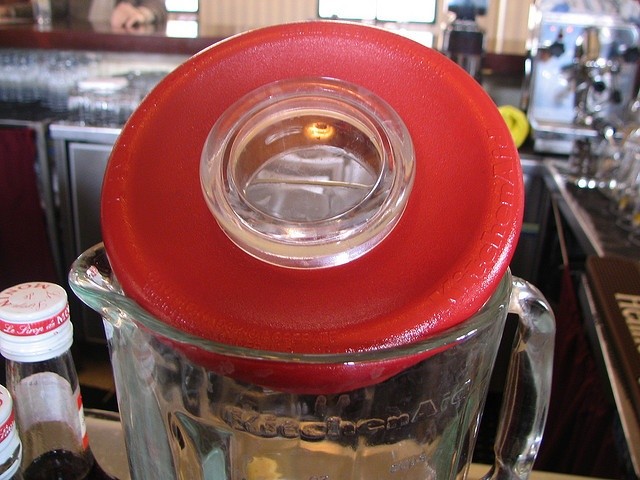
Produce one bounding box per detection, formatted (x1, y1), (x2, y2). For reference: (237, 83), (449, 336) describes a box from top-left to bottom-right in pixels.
(0, 117), (62, 295)
(46, 125), (123, 345)
(494, 173), (640, 480)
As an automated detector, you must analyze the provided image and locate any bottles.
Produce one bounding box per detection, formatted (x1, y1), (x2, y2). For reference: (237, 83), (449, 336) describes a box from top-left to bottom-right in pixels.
(0, 384), (23, 480)
(0, 282), (93, 480)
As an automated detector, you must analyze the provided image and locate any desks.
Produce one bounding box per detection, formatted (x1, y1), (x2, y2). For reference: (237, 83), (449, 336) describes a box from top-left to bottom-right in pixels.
(82, 411), (600, 480)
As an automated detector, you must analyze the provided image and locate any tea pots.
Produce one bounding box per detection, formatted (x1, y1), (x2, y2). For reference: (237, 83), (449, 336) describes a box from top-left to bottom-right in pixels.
(67, 20), (558, 480)
